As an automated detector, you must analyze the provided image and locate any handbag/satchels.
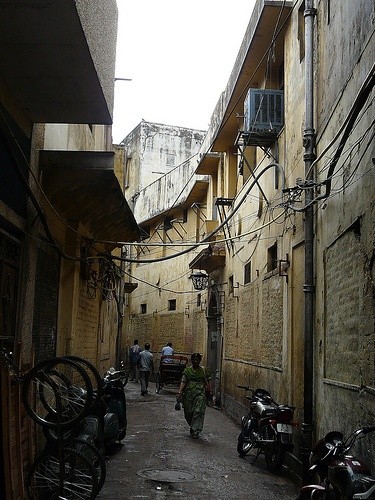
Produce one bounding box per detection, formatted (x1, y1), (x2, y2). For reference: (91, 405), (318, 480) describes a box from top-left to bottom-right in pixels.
(175, 399), (181, 410)
(131, 352), (137, 364)
(201, 366), (213, 400)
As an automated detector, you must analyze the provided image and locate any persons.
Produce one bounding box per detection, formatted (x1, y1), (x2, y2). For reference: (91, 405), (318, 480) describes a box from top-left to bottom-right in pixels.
(176, 352), (211, 436)
(128, 339), (142, 381)
(161, 342), (174, 363)
(138, 343), (154, 395)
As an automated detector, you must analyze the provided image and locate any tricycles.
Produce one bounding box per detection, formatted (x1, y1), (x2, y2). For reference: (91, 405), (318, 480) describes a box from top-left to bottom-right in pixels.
(155, 354), (189, 394)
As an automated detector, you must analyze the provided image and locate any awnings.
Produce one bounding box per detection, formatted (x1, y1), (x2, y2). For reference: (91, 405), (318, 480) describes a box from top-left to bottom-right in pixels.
(40, 149), (140, 251)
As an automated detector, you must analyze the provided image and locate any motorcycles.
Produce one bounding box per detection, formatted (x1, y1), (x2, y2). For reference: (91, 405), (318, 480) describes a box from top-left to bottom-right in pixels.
(237, 385), (296, 472)
(79, 360), (130, 451)
(297, 426), (375, 500)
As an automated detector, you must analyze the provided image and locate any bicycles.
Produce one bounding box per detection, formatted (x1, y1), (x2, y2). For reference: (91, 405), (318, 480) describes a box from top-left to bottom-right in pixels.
(0, 347), (107, 500)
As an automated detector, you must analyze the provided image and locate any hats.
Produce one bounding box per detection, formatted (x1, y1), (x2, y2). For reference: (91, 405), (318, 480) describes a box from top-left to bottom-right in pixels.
(145, 343), (151, 347)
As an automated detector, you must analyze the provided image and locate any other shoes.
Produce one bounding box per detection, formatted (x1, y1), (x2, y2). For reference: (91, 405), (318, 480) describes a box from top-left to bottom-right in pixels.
(193, 431), (198, 439)
(190, 428), (194, 436)
